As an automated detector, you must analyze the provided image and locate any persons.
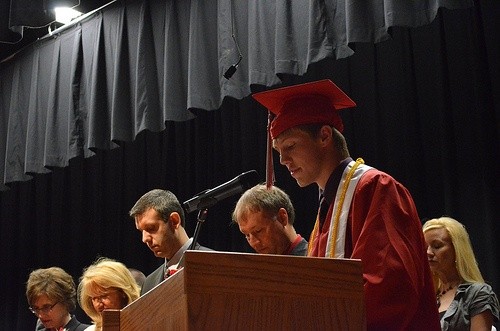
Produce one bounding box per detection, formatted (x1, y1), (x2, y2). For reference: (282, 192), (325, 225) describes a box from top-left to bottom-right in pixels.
(232, 184), (309, 256)
(129, 269), (147, 288)
(422, 216), (500, 331)
(78, 257), (145, 331)
(251, 79), (436, 331)
(129, 189), (219, 297)
(25, 265), (88, 331)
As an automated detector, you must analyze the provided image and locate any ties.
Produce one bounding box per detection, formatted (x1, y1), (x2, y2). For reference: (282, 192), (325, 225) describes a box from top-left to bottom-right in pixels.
(59, 326), (63, 331)
(168, 269), (177, 275)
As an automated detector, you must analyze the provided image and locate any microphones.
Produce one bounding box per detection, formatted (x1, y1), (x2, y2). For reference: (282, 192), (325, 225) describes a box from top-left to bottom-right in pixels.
(184, 170), (258, 213)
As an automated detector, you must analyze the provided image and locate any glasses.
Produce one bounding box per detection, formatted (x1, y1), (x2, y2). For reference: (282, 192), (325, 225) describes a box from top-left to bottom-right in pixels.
(29, 300), (59, 315)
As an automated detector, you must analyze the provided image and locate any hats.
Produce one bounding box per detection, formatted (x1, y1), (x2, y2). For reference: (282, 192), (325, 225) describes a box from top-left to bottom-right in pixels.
(252, 79), (357, 139)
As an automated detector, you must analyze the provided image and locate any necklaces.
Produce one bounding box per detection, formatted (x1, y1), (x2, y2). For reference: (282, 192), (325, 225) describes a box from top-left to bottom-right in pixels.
(435, 282), (460, 308)
